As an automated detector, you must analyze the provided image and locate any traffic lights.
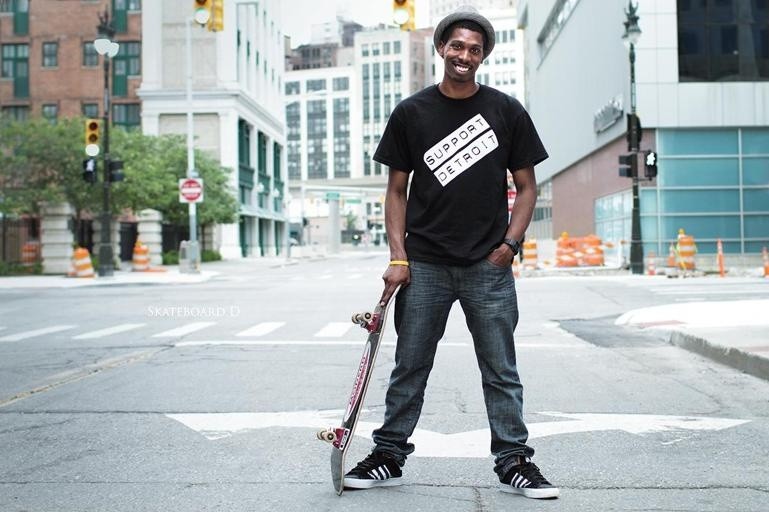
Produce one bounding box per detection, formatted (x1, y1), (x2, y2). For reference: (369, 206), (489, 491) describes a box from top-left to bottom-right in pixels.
(194, 0), (213, 26)
(85, 120), (102, 158)
(641, 149), (656, 178)
(83, 160), (97, 183)
(394, 0), (414, 28)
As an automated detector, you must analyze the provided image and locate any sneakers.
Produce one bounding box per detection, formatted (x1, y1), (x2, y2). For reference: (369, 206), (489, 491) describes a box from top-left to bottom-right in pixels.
(343, 452), (404, 490)
(501, 462), (559, 500)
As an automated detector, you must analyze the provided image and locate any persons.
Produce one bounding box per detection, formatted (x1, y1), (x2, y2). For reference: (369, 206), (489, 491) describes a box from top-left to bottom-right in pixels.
(342, 7), (562, 500)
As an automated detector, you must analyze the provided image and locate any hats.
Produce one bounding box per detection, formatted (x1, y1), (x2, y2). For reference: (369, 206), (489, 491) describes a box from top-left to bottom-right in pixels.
(434, 5), (496, 64)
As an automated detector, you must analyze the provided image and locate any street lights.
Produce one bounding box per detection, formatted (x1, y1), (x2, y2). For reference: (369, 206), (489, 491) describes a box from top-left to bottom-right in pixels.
(620, 1), (642, 272)
(93, 4), (120, 277)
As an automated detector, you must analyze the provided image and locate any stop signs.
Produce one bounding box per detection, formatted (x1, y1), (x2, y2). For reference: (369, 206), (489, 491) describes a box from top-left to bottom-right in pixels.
(179, 178), (204, 202)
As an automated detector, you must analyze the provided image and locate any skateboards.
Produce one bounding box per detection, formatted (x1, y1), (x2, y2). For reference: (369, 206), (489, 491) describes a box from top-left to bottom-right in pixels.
(317, 283), (402, 496)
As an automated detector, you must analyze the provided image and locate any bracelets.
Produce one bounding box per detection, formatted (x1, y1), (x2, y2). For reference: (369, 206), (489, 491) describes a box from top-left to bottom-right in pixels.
(389, 260), (409, 266)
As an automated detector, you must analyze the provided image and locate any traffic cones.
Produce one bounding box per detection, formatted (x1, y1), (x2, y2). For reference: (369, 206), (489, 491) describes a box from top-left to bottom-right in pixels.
(645, 252), (656, 276)
(669, 244), (677, 267)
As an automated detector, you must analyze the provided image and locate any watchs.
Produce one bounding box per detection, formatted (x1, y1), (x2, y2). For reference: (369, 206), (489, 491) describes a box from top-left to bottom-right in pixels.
(502, 239), (521, 254)
